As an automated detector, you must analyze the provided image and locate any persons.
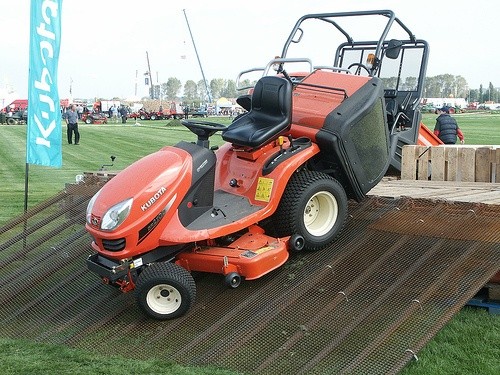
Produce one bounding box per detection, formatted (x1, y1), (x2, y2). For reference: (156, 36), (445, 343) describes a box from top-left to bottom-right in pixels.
(433, 108), (465, 146)
(109, 105), (133, 123)
(183, 106), (190, 119)
(65, 103), (81, 146)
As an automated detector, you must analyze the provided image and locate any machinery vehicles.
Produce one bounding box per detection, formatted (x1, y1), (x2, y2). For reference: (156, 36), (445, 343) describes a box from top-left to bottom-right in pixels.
(85, 58), (391, 320)
(418, 99), (500, 115)
(236, 10), (446, 177)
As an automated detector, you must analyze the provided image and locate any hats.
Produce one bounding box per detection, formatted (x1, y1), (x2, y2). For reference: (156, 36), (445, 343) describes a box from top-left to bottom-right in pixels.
(438, 107), (448, 111)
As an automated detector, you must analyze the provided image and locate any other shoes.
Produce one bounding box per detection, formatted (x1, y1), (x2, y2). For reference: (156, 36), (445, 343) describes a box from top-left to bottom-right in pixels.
(69, 143), (72, 144)
(75, 144), (80, 145)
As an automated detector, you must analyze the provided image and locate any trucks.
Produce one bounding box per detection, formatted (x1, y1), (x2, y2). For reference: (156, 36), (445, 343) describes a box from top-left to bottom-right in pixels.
(0, 97), (244, 125)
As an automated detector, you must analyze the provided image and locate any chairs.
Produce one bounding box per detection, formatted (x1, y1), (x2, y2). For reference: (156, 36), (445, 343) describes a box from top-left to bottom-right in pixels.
(222, 76), (292, 149)
